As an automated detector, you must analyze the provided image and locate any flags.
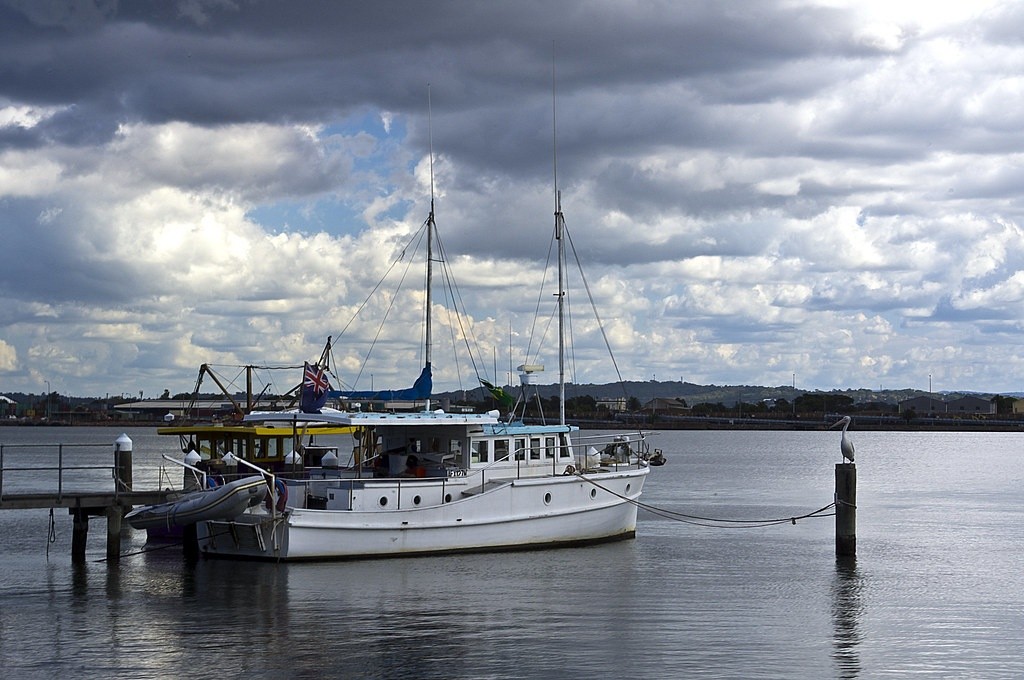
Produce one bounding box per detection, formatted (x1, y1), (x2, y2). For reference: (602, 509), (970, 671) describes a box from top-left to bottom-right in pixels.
(300, 362), (330, 415)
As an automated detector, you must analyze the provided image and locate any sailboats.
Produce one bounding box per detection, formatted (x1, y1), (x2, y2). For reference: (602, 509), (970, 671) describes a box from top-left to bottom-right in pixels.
(125, 37), (665, 563)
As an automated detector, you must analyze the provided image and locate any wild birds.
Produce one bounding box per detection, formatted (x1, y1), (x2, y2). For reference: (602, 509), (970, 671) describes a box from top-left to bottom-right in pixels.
(829, 416), (855, 465)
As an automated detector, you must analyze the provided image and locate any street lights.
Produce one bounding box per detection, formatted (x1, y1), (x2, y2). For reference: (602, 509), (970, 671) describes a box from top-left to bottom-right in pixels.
(929, 373), (933, 414)
(507, 372), (510, 386)
(43, 379), (51, 419)
(792, 373), (799, 412)
(370, 373), (374, 391)
(126, 393), (132, 418)
